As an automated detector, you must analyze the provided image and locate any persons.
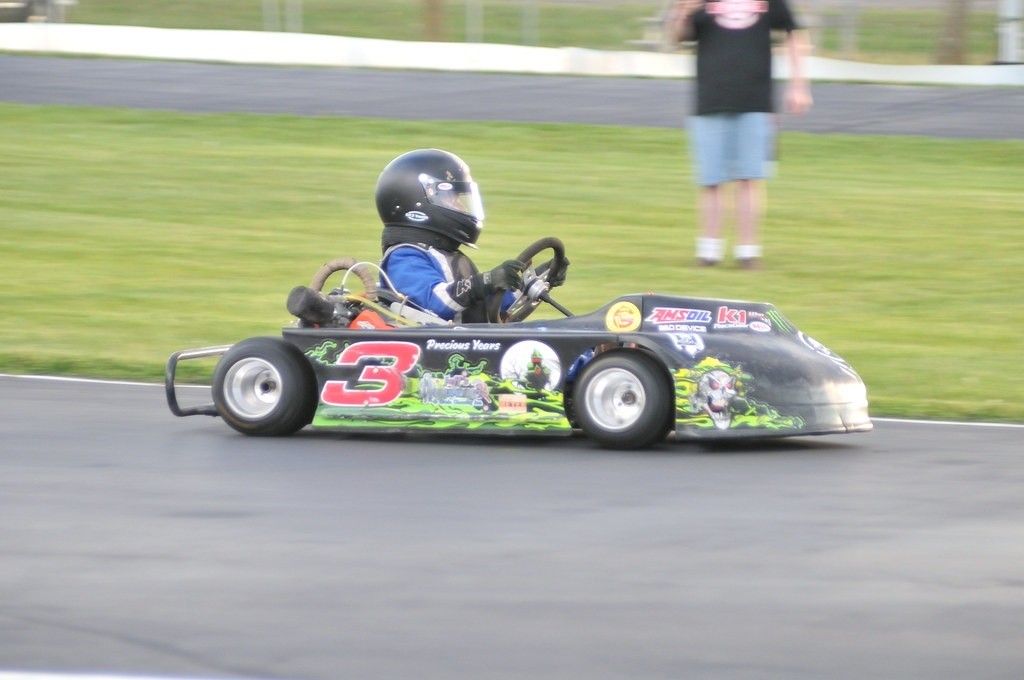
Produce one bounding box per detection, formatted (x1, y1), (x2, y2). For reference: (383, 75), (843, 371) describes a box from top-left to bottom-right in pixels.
(374, 148), (570, 325)
(663, 0), (811, 267)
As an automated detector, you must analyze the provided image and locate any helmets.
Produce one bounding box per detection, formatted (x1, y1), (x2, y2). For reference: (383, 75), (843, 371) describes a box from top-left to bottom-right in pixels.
(375, 148), (484, 250)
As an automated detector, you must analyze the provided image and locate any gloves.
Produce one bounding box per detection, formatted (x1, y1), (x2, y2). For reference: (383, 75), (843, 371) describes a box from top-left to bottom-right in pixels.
(478, 259), (532, 292)
(535, 257), (569, 288)
